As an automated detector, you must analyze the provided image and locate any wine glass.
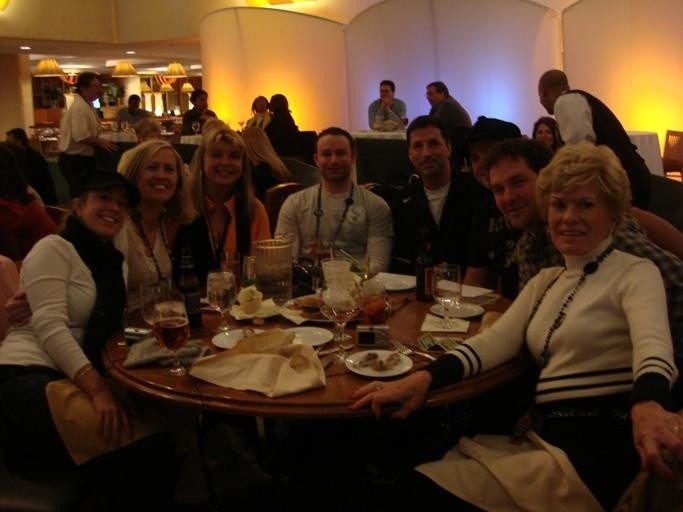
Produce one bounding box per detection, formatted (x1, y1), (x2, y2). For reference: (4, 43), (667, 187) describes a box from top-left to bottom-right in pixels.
(137, 281), (171, 326)
(317, 277), (362, 352)
(111, 122), (117, 137)
(430, 261), (463, 327)
(122, 120), (128, 135)
(206, 270), (238, 331)
(192, 122), (201, 136)
(152, 302), (192, 377)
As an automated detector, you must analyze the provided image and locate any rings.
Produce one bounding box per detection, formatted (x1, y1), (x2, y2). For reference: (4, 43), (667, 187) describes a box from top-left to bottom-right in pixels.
(672, 426), (679, 435)
(374, 382), (380, 389)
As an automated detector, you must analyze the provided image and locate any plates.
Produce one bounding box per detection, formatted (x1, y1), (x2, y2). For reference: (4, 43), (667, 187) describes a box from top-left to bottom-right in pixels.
(344, 349), (414, 378)
(284, 326), (335, 347)
(363, 271), (417, 292)
(211, 326), (266, 350)
(430, 302), (485, 318)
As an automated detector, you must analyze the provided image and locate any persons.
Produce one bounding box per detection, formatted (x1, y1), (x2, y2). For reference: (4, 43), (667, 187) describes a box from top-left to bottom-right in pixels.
(265, 94), (299, 138)
(167, 126), (282, 449)
(481, 136), (683, 303)
(135, 117), (160, 142)
(538, 70), (652, 212)
(243, 96), (274, 132)
(0, 141), (61, 263)
(533, 118), (563, 155)
(273, 128), (393, 277)
(116, 95), (152, 125)
(368, 80), (407, 131)
(371, 116), (500, 276)
(466, 114), (522, 289)
(182, 88), (216, 135)
(0, 173), (191, 512)
(348, 142), (683, 512)
(237, 126), (293, 204)
(59, 72), (118, 199)
(5, 128), (57, 205)
(202, 118), (225, 136)
(426, 81), (469, 127)
(4, 140), (275, 512)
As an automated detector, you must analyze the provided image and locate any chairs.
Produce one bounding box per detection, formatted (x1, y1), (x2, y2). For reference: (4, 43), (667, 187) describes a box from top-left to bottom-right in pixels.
(267, 182), (299, 225)
(103, 276), (528, 418)
(661, 129), (683, 174)
(616, 410), (683, 512)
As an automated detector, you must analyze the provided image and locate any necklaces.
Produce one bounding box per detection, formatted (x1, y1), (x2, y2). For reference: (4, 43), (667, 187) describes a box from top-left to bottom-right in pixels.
(511, 244), (615, 445)
(310, 181), (355, 257)
(132, 207), (177, 298)
(200, 189), (232, 271)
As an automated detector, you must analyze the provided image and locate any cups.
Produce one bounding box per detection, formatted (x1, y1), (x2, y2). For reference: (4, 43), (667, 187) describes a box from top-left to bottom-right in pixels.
(320, 257), (353, 285)
(249, 238), (290, 306)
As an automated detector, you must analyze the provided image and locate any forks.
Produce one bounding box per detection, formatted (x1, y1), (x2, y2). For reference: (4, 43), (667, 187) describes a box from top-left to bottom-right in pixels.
(392, 338), (437, 362)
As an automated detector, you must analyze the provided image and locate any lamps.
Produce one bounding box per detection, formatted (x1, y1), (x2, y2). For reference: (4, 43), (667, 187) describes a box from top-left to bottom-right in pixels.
(160, 82), (172, 92)
(111, 58), (136, 78)
(32, 57), (66, 79)
(163, 54), (187, 79)
(182, 81), (194, 95)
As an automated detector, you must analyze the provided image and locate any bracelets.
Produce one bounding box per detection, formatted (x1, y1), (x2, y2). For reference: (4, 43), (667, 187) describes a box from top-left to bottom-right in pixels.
(76, 365), (95, 386)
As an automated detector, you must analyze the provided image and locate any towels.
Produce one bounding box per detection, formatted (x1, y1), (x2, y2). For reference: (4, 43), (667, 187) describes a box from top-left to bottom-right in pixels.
(187, 329), (326, 400)
(415, 427), (603, 512)
(42, 377), (165, 469)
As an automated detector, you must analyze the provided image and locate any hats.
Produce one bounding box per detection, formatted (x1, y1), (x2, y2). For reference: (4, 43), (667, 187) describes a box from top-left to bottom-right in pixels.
(80, 168), (141, 209)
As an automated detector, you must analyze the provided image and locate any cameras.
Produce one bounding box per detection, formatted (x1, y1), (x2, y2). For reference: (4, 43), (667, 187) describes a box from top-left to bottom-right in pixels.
(123, 327), (152, 349)
(357, 323), (390, 348)
